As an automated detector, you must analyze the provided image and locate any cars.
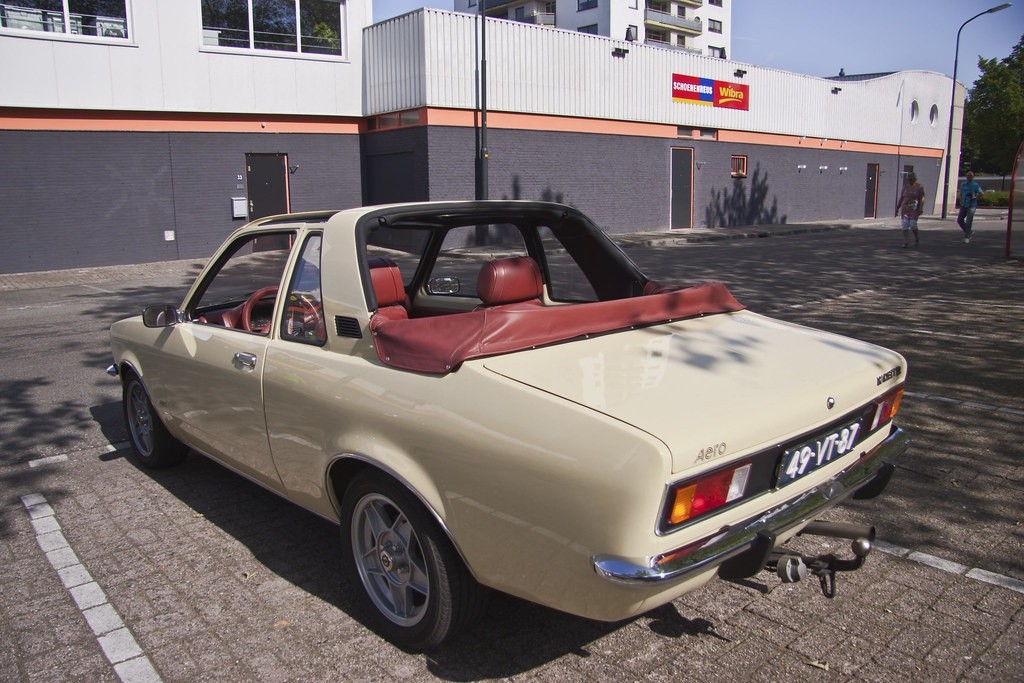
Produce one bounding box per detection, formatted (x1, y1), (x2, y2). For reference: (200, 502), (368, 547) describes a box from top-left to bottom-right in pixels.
(105, 200), (911, 654)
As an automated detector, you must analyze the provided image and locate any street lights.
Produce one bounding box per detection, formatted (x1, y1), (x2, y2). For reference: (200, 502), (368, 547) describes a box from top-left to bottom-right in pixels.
(941, 3), (1012, 218)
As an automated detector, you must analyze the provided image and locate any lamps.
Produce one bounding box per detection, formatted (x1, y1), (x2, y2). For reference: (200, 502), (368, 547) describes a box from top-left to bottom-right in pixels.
(612, 46), (630, 55)
(817, 138), (830, 147)
(733, 69), (747, 75)
(256, 121), (272, 130)
(796, 136), (810, 145)
(838, 138), (850, 147)
(830, 87), (842, 94)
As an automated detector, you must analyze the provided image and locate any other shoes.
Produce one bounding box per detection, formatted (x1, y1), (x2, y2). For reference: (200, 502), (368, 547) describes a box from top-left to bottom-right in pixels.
(915, 237), (920, 247)
(900, 242), (908, 249)
(969, 231), (975, 240)
(964, 238), (970, 243)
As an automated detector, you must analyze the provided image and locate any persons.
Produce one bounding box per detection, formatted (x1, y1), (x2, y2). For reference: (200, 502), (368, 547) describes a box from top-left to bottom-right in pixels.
(895, 172), (925, 247)
(957, 172), (984, 243)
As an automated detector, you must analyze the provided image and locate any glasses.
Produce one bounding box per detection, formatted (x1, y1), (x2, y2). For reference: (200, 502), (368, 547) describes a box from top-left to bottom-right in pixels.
(907, 178), (914, 181)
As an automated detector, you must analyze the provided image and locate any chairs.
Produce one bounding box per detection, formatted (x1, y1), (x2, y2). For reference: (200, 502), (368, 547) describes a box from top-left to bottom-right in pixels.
(365, 254), (411, 323)
(459, 253), (561, 313)
